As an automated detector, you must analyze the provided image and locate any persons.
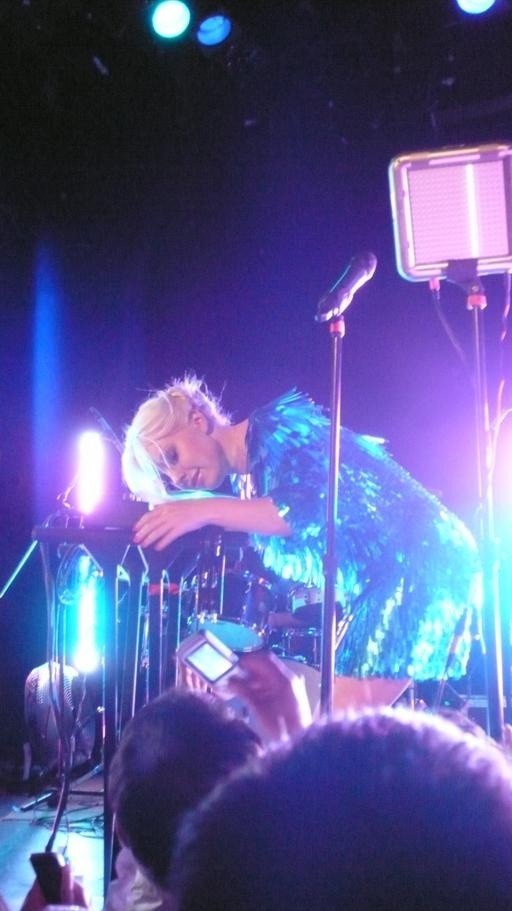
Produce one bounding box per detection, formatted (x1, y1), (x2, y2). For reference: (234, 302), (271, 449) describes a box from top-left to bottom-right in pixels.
(171, 709), (512, 911)
(0, 864), (89, 911)
(120, 366), (487, 722)
(105, 645), (314, 909)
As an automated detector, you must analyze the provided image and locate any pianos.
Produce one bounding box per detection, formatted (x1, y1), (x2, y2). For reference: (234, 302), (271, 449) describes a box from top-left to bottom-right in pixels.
(85, 495), (221, 556)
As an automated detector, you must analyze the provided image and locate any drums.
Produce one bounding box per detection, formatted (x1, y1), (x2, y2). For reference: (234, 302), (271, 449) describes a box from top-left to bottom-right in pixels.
(188, 565), (275, 655)
(288, 584), (343, 622)
(281, 628), (324, 668)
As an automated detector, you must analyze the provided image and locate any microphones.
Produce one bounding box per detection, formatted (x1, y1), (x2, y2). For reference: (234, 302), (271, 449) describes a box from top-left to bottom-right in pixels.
(315, 249), (378, 324)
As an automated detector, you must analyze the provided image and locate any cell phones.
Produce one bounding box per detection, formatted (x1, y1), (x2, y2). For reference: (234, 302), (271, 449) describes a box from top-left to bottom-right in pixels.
(30, 853), (66, 905)
(175, 628), (250, 702)
